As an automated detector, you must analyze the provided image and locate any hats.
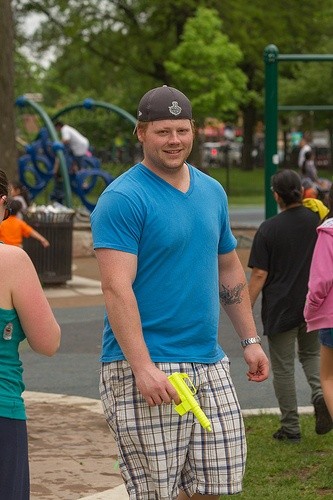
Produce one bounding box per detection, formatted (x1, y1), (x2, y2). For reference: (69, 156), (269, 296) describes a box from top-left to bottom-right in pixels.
(132, 84), (193, 134)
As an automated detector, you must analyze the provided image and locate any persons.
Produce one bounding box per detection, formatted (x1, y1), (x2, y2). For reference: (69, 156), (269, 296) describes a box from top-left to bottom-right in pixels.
(0, 200), (50, 249)
(9, 181), (30, 220)
(0, 169), (62, 500)
(303, 183), (333, 422)
(90, 84), (270, 500)
(297, 137), (318, 182)
(50, 157), (78, 204)
(299, 178), (333, 223)
(246, 169), (332, 441)
(55, 122), (91, 171)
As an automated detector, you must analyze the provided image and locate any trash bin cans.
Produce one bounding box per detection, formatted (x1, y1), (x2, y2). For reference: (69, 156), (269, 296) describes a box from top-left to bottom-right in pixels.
(22, 203), (76, 288)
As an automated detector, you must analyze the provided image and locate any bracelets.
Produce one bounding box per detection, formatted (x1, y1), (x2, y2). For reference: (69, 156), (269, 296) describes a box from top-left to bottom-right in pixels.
(240, 336), (263, 347)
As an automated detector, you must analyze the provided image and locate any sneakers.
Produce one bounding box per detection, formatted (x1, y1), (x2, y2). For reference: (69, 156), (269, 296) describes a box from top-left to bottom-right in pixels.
(314, 395), (333, 434)
(273, 429), (302, 440)
